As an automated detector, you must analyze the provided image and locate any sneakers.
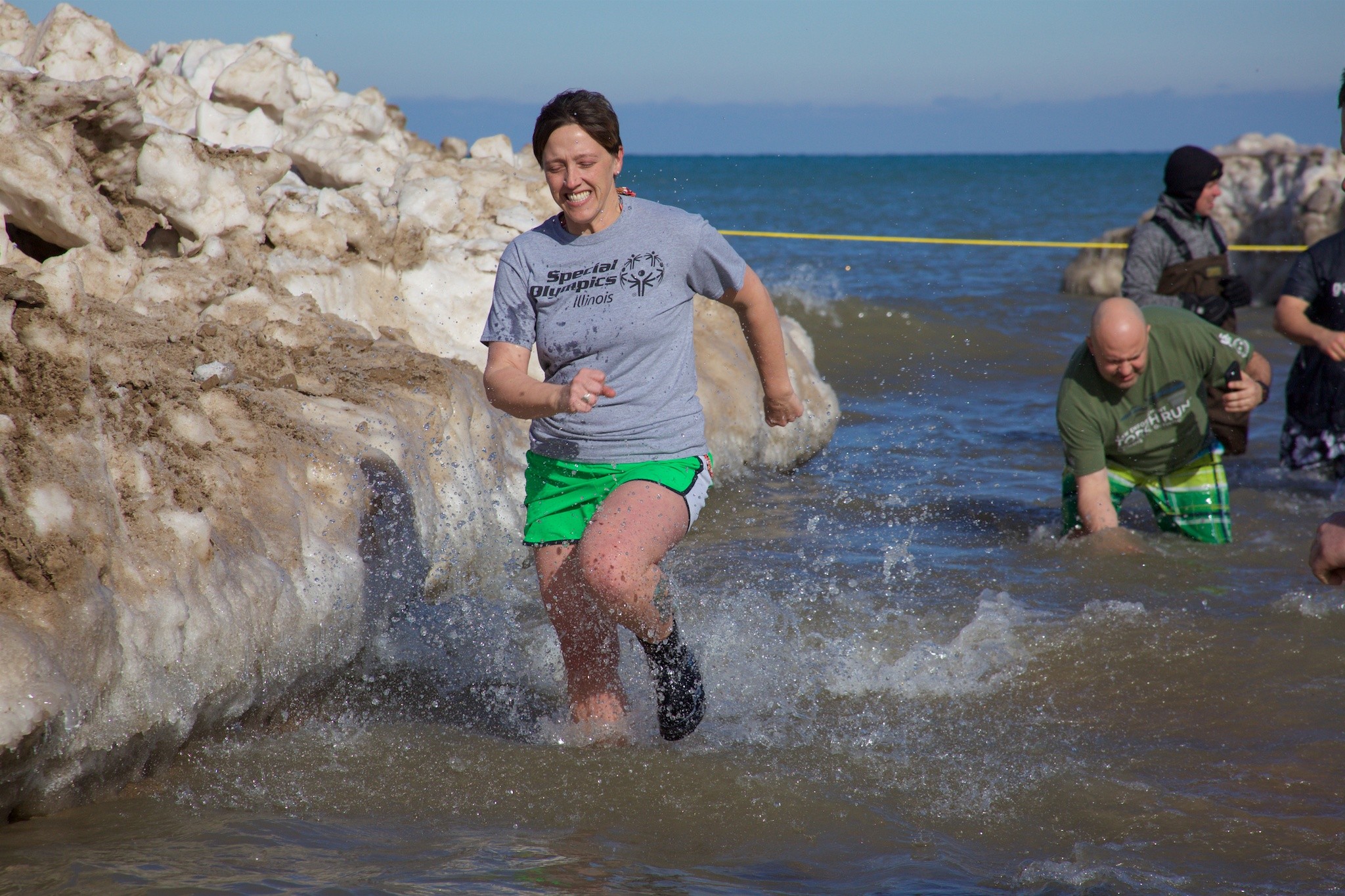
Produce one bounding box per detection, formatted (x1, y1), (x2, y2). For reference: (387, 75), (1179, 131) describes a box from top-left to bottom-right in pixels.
(636, 620), (705, 743)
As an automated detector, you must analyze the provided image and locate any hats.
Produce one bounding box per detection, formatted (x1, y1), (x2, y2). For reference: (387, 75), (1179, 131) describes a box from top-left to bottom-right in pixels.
(1164, 146), (1223, 214)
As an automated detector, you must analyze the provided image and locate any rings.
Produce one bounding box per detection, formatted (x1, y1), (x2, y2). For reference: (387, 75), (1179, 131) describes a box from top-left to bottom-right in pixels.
(581, 393), (591, 402)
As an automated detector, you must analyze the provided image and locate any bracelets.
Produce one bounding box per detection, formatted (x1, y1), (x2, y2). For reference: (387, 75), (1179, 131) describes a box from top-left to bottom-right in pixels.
(1257, 380), (1270, 406)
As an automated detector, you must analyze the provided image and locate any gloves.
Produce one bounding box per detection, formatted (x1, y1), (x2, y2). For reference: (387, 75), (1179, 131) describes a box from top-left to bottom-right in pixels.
(1218, 275), (1252, 307)
(1181, 293), (1232, 328)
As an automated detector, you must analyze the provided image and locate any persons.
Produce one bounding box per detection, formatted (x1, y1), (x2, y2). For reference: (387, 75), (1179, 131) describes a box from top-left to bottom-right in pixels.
(1055, 296), (1272, 545)
(476, 85), (803, 742)
(1272, 69), (1345, 588)
(1122, 146), (1256, 458)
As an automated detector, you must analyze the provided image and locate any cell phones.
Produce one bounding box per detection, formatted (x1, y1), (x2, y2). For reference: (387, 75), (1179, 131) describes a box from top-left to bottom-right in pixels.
(1226, 362), (1244, 396)
